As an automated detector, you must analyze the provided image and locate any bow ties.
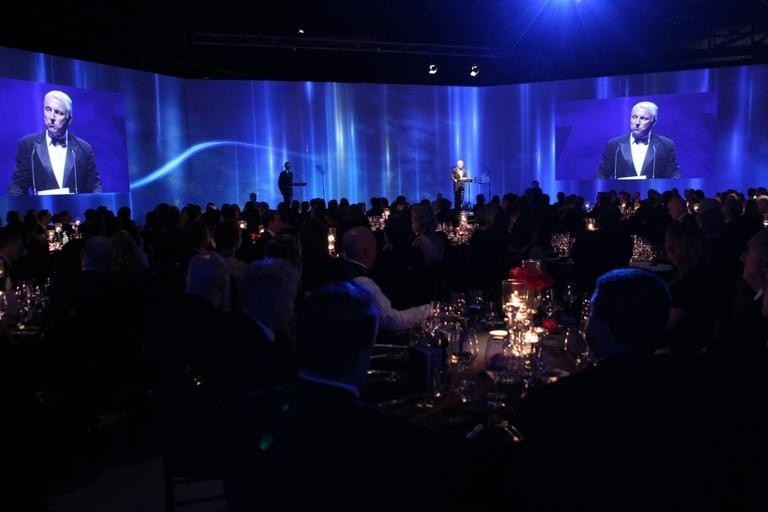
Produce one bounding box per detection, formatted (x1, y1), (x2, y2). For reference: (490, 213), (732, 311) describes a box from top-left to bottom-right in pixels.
(635, 136), (647, 145)
(51, 136), (66, 147)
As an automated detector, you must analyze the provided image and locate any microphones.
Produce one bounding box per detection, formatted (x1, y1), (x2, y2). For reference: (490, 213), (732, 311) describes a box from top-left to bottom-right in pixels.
(69, 145), (78, 195)
(651, 143), (656, 178)
(31, 143), (38, 195)
(614, 144), (621, 180)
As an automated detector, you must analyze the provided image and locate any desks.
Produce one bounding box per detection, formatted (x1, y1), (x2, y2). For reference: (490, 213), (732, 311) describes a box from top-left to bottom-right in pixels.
(362, 315), (593, 447)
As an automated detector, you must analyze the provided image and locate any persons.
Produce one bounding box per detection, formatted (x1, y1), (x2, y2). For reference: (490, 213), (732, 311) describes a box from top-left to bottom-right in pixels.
(277, 161), (293, 205)
(6, 89), (104, 197)
(449, 159), (469, 214)
(594, 101), (681, 180)
